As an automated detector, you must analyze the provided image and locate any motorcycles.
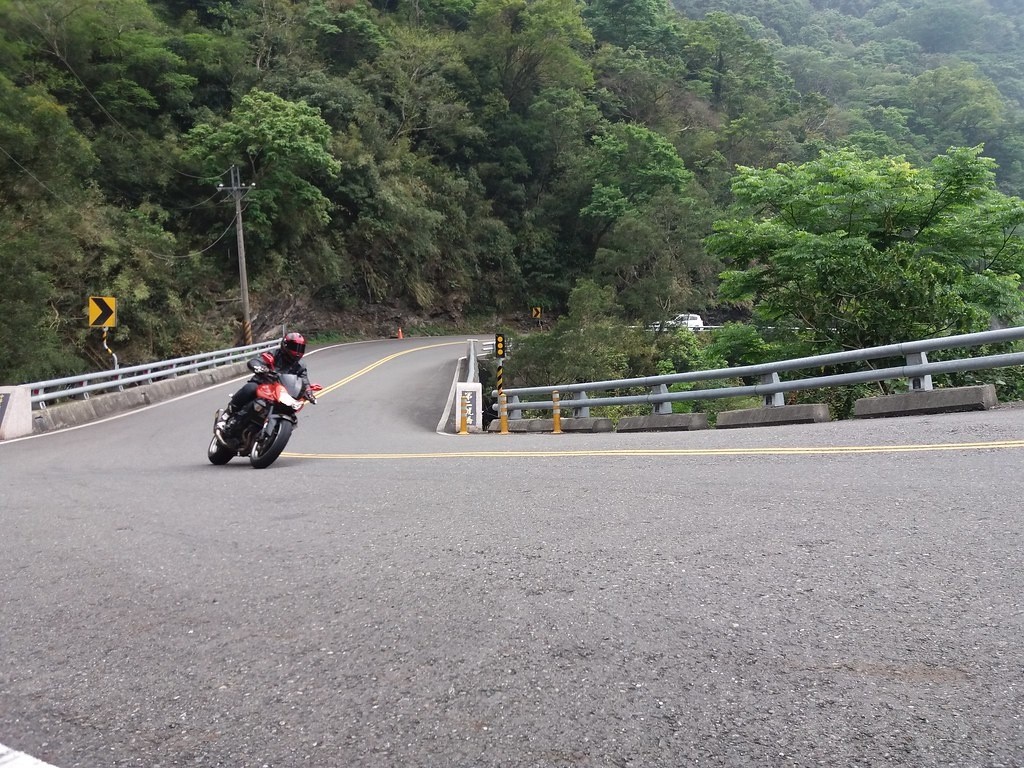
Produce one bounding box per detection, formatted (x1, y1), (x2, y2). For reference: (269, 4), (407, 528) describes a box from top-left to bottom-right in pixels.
(208, 352), (322, 469)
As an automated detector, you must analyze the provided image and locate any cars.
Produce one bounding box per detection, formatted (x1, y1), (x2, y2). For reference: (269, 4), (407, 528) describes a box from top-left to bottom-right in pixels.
(648, 312), (704, 332)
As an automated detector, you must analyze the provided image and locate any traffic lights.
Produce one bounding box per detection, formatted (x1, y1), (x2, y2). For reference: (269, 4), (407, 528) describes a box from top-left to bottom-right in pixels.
(496, 333), (505, 358)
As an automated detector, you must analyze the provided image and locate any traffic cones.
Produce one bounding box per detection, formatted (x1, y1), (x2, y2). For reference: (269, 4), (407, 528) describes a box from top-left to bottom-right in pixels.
(397, 327), (403, 339)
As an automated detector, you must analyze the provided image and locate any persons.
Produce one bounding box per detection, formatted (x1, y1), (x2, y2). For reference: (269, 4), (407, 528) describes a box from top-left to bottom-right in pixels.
(221, 331), (315, 428)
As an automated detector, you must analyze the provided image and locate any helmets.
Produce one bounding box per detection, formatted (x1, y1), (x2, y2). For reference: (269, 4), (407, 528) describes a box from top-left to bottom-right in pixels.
(281, 333), (306, 363)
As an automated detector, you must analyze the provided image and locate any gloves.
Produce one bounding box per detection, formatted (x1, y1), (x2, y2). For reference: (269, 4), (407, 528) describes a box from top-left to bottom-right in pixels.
(259, 365), (269, 378)
(308, 395), (316, 404)
